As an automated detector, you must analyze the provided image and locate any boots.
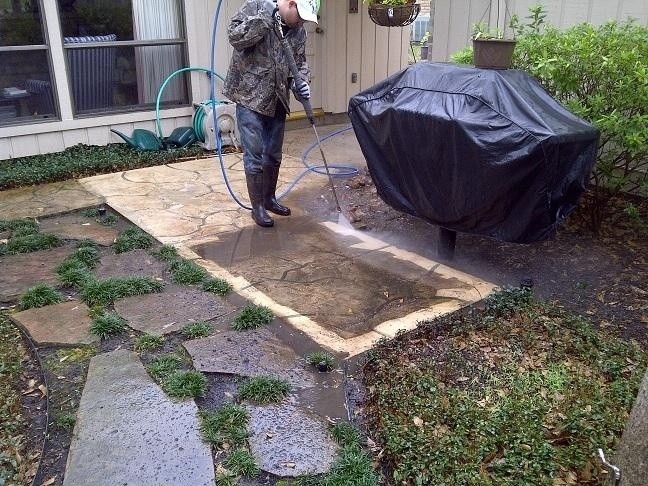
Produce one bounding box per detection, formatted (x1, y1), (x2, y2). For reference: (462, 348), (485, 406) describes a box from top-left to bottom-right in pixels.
(245, 174), (274, 227)
(263, 168), (291, 215)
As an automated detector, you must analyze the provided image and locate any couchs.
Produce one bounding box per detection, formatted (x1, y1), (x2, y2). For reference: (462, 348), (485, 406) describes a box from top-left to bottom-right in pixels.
(26, 33), (116, 114)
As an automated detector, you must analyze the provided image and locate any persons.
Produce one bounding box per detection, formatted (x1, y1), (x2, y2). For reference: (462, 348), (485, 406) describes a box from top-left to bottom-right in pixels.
(222, 0), (320, 227)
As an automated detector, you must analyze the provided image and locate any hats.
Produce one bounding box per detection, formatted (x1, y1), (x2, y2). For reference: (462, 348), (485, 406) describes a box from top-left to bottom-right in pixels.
(293, 0), (322, 26)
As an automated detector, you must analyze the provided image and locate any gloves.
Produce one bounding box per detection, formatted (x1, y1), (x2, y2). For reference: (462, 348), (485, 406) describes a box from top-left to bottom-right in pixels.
(292, 80), (311, 101)
(261, 0), (281, 25)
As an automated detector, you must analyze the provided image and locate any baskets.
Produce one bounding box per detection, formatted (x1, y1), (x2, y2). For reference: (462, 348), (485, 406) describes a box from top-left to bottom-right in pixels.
(368, 3), (421, 28)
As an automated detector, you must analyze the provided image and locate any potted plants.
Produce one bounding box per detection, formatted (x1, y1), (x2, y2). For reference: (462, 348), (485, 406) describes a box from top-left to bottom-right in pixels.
(470, 19), (518, 70)
(364, 0), (421, 27)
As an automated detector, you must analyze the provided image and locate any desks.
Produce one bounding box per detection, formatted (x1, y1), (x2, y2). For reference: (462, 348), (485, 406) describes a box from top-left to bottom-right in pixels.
(0, 84), (39, 118)
